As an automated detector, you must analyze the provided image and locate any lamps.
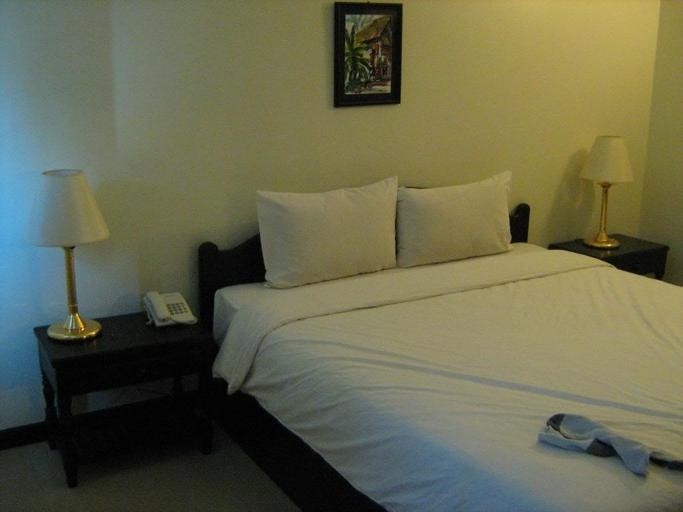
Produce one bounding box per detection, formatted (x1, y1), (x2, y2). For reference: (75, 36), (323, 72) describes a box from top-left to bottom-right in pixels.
(579, 135), (634, 251)
(25, 168), (110, 341)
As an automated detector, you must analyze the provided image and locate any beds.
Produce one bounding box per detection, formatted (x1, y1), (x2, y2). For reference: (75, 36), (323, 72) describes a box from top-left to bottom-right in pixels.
(197, 186), (683, 512)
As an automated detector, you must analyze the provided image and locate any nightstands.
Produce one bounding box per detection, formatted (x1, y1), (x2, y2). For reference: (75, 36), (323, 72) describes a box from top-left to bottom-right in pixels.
(548, 233), (671, 281)
(33, 310), (212, 489)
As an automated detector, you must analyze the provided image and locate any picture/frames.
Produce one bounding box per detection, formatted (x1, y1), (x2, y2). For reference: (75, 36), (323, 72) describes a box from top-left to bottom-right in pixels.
(333, 1), (404, 107)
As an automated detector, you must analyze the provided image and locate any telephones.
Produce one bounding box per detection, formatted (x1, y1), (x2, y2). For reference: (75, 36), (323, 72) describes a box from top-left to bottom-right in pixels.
(143, 290), (199, 328)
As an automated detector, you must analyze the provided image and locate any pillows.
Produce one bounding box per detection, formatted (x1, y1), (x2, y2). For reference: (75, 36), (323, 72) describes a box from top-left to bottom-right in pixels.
(255, 174), (398, 290)
(396, 169), (513, 268)
